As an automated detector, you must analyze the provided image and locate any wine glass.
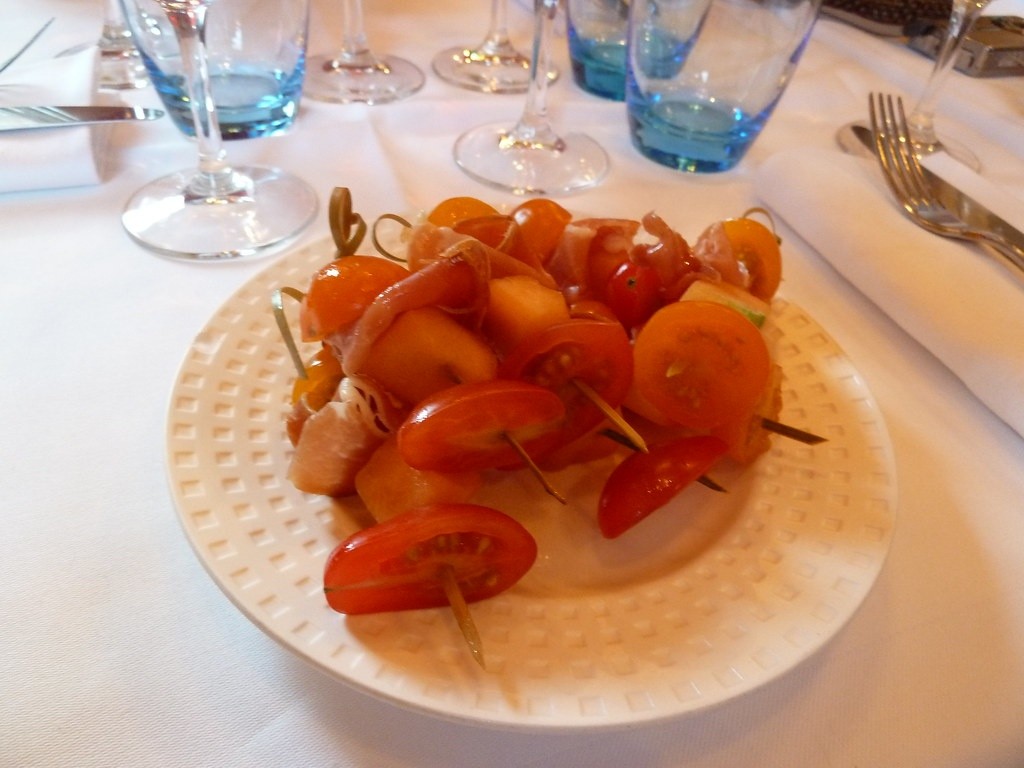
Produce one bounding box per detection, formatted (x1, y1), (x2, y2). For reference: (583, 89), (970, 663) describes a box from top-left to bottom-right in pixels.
(55, 0), (152, 94)
(122, 0), (319, 260)
(454, 0), (611, 195)
(838, 0), (993, 175)
(432, 0), (559, 93)
(301, 0), (425, 104)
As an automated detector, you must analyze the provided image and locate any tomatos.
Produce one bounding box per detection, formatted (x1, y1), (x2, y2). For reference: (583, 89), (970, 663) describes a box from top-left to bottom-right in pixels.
(277, 195), (780, 613)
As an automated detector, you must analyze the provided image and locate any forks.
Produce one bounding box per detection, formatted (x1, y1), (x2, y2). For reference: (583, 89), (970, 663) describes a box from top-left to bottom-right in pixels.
(868, 93), (1024, 270)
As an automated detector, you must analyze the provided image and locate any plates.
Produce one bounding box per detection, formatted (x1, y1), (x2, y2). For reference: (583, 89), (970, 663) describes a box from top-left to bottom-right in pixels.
(162, 213), (898, 730)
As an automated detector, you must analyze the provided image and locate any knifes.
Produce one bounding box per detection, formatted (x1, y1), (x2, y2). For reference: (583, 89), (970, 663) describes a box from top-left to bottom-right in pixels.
(851, 126), (1024, 282)
(0, 106), (165, 130)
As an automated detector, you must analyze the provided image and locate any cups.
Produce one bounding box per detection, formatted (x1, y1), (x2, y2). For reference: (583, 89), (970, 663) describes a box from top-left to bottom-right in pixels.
(120, 0), (308, 139)
(626, 0), (822, 172)
(565, 0), (714, 102)
